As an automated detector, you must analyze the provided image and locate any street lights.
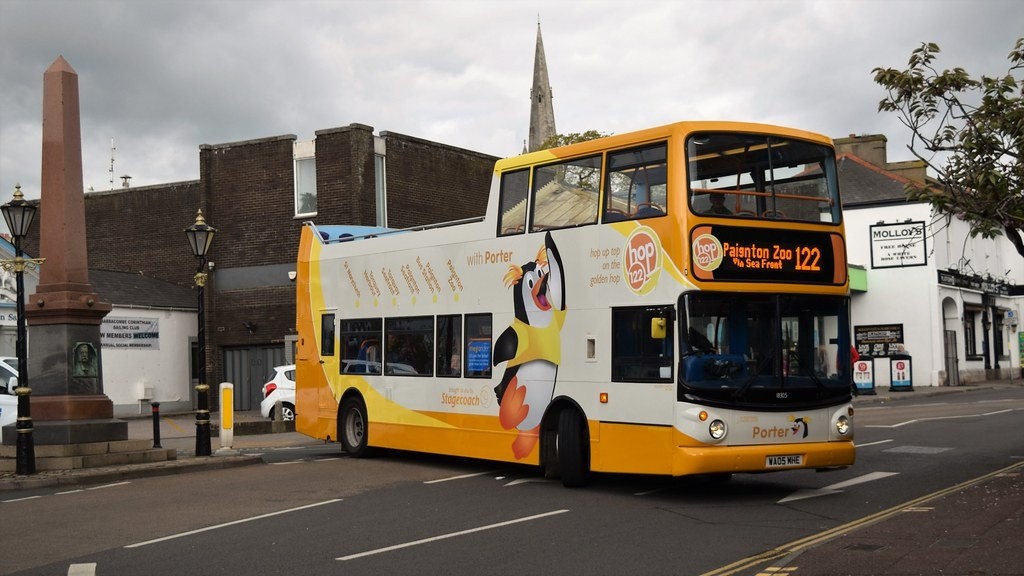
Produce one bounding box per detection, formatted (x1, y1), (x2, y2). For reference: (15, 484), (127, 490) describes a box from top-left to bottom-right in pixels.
(184, 208), (220, 456)
(1, 182), (47, 477)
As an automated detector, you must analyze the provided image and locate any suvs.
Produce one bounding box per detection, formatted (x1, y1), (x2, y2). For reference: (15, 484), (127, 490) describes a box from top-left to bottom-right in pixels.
(259, 364), (297, 421)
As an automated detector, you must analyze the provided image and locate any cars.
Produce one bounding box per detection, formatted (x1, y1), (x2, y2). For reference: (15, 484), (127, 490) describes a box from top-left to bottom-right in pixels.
(0, 357), (20, 445)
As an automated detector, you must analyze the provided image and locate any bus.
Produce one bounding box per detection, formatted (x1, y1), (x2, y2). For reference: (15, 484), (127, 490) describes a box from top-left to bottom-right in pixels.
(291, 119), (855, 490)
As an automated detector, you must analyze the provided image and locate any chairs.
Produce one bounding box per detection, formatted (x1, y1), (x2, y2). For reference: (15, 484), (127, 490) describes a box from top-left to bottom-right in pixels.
(633, 202), (664, 219)
(733, 209), (758, 217)
(356, 339), (378, 374)
(594, 209), (629, 222)
(762, 210), (786, 218)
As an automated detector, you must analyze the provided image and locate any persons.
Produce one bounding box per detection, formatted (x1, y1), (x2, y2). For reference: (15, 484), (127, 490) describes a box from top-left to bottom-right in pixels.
(439, 353), (460, 377)
(387, 333), (403, 364)
(706, 188), (733, 216)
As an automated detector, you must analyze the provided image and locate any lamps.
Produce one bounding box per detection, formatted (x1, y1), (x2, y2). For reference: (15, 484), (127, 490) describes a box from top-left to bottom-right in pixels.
(243, 321), (257, 334)
